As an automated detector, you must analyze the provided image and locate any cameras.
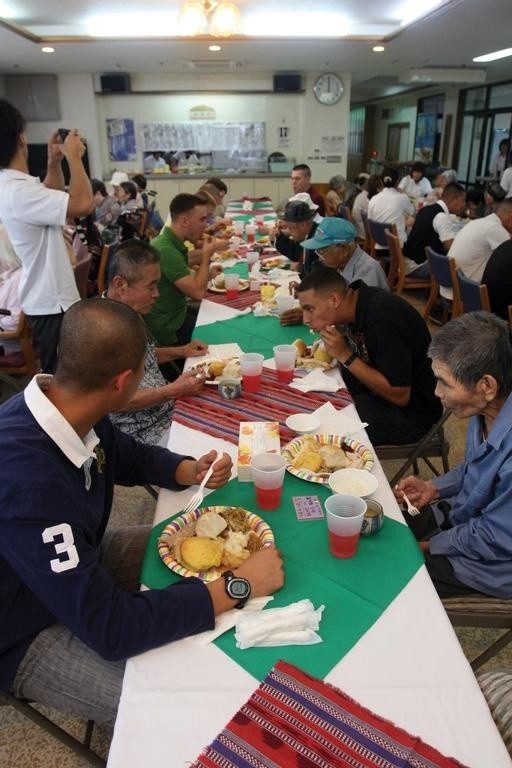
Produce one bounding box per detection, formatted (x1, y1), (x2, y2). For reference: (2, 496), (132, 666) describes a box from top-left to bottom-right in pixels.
(58, 128), (71, 144)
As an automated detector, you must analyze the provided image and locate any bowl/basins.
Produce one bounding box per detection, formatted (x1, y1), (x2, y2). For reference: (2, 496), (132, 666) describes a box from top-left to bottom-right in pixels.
(325, 468), (379, 497)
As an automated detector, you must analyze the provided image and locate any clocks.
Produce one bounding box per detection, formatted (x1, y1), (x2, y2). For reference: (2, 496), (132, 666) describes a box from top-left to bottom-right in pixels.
(313, 70), (345, 106)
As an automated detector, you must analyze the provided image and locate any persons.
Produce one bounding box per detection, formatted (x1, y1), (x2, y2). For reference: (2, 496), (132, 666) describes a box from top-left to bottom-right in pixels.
(258, 164), (392, 328)
(144, 178), (233, 376)
(0, 297), (284, 727)
(102, 239), (206, 446)
(1, 97), (94, 375)
(324, 159), (511, 321)
(64, 172), (157, 295)
(1, 226), (20, 354)
(494, 137), (509, 180)
(143, 151), (201, 174)
(297, 266), (443, 447)
(392, 311), (511, 598)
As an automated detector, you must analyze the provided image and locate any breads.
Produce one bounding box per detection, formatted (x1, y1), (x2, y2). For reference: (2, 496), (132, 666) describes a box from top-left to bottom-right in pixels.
(293, 339), (307, 356)
(208, 362), (226, 377)
(296, 436), (347, 473)
(314, 348), (332, 364)
(178, 536), (223, 573)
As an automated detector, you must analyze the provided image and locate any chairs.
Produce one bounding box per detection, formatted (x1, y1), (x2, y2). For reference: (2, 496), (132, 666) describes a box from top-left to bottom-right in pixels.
(0, 198), (163, 393)
(436, 593), (512, 672)
(0, 687), (111, 767)
(373, 405), (456, 487)
(305, 162), (512, 330)
(140, 483), (161, 503)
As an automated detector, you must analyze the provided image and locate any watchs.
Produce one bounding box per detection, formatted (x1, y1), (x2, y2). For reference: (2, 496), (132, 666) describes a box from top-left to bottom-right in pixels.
(221, 570), (251, 609)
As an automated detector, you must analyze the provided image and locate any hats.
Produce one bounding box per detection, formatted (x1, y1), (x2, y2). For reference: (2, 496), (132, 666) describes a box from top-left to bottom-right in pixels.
(108, 171), (129, 186)
(299, 217), (357, 250)
(278, 192), (320, 222)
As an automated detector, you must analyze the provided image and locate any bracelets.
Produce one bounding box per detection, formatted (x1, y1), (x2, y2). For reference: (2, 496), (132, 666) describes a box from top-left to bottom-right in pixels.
(343, 353), (357, 368)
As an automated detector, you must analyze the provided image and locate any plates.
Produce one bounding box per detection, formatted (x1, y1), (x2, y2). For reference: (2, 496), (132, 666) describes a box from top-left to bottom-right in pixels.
(283, 435), (375, 485)
(143, 165), (226, 176)
(212, 241), (287, 271)
(187, 359), (243, 385)
(157, 506), (276, 583)
(285, 413), (321, 433)
(297, 345), (337, 371)
(207, 279), (248, 292)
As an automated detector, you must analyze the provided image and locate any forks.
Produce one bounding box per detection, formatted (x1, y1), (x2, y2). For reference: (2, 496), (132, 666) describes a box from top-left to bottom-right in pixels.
(182, 448), (225, 513)
(394, 484), (421, 517)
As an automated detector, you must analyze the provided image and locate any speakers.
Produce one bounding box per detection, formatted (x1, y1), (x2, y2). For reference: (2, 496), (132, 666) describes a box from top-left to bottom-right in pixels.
(273, 75), (301, 93)
(100, 75), (126, 94)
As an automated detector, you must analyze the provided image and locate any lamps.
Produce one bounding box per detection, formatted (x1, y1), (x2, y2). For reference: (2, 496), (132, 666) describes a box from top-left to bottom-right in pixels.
(176, 0), (242, 38)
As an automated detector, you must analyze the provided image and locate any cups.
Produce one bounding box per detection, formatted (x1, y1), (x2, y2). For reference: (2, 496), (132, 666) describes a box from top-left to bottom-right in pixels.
(258, 285), (274, 303)
(238, 352), (265, 392)
(276, 294), (295, 313)
(273, 344), (298, 382)
(251, 452), (286, 511)
(324, 493), (367, 559)
(256, 214), (264, 226)
(224, 273), (240, 300)
(246, 225), (256, 243)
(361, 499), (385, 537)
(418, 198), (423, 208)
(234, 221), (244, 237)
